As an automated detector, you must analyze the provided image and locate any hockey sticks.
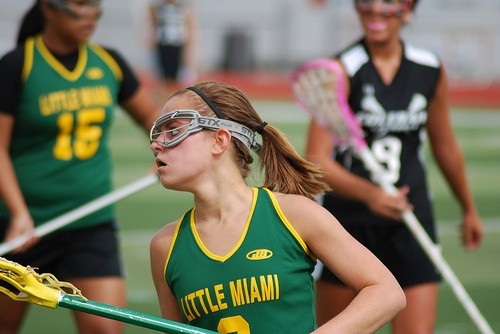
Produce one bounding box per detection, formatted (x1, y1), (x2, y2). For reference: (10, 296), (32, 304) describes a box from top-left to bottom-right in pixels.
(0, 170), (161, 259)
(288, 58), (495, 334)
(0, 255), (219, 334)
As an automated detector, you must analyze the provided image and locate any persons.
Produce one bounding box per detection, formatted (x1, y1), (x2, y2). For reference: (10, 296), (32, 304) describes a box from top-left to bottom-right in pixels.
(148, 83), (407, 334)
(306, 0), (483, 333)
(1, 1), (164, 334)
(150, 0), (193, 88)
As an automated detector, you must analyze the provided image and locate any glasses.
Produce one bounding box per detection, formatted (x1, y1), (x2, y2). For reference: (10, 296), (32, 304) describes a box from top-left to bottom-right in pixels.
(148, 109), (258, 149)
